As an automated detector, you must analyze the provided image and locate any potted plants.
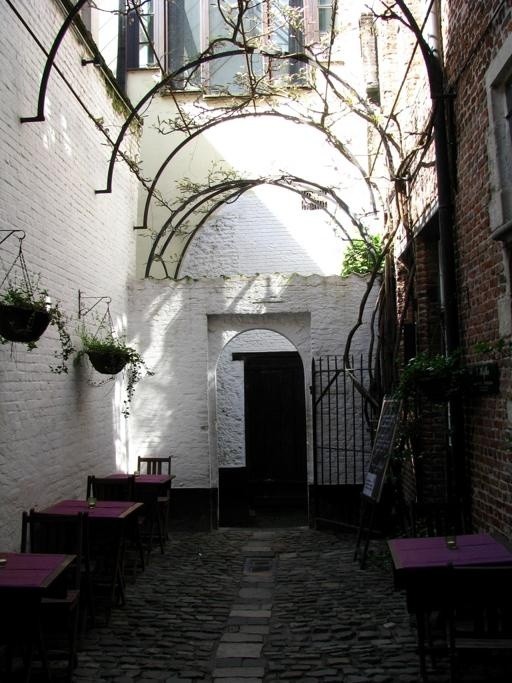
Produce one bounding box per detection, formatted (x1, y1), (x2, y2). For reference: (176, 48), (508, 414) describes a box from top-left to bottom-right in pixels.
(378, 345), (466, 466)
(0, 270), (155, 417)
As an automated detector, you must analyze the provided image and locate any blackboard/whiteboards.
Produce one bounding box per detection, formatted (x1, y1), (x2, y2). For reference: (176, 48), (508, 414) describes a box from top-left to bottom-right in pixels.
(363, 399), (403, 501)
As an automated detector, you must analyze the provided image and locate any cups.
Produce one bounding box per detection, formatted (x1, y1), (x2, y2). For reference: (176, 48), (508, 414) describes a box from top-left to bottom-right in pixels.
(134, 471), (140, 478)
(88, 497), (97, 508)
(446, 535), (457, 549)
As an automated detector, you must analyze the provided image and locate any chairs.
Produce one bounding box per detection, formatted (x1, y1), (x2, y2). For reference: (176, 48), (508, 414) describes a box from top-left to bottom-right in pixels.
(0, 456), (171, 682)
(398, 494), (511, 682)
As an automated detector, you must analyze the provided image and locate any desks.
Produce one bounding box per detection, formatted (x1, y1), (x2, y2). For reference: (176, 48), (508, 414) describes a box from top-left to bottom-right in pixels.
(386, 533), (512, 682)
(104, 473), (180, 554)
